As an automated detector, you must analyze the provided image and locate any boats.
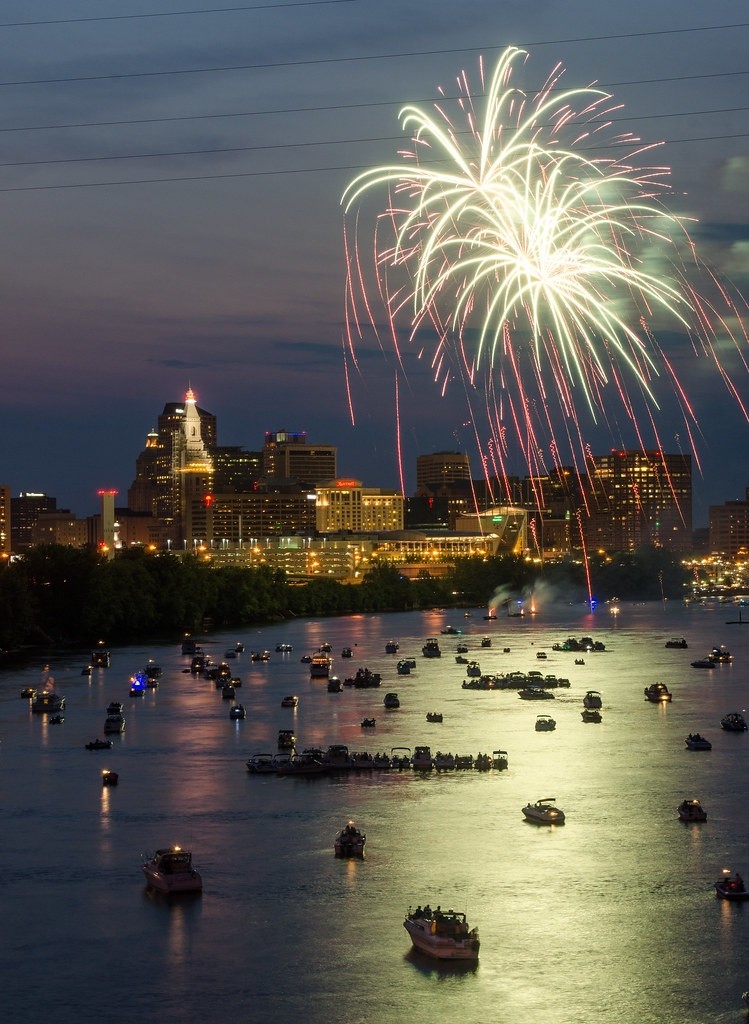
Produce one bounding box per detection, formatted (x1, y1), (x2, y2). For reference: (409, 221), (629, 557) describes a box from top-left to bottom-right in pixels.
(249, 612), (607, 708)
(665, 638), (688, 648)
(143, 847), (203, 894)
(402, 903), (480, 960)
(704, 646), (736, 662)
(247, 730), (513, 777)
(720, 713), (747, 732)
(103, 770), (119, 785)
(522, 797), (566, 824)
(714, 876), (749, 900)
(684, 733), (712, 750)
(691, 660), (716, 669)
(360, 718), (376, 727)
(536, 714), (556, 731)
(427, 712), (443, 722)
(643, 682), (672, 701)
(678, 799), (707, 821)
(18, 637), (246, 753)
(334, 824), (367, 859)
(581, 710), (603, 724)
